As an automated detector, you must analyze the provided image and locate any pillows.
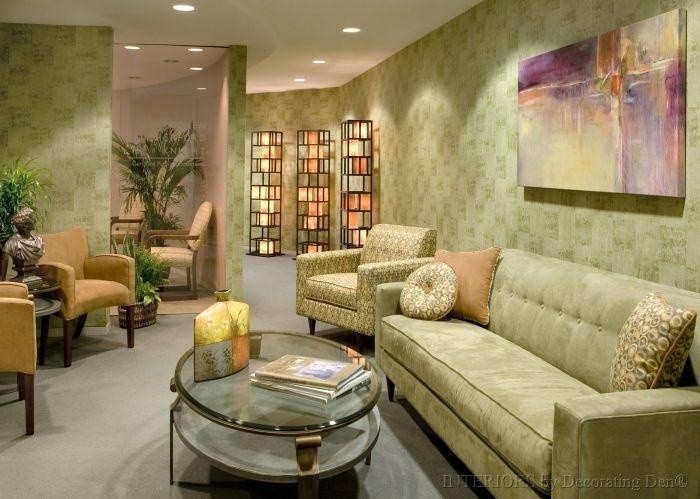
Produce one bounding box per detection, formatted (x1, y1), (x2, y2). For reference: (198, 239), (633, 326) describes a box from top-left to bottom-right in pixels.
(434, 241), (502, 330)
(604, 292), (698, 395)
(397, 261), (461, 321)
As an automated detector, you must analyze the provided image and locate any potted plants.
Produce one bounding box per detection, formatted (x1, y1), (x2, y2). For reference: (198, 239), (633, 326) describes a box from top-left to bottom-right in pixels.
(111, 121), (207, 291)
(107, 224), (178, 328)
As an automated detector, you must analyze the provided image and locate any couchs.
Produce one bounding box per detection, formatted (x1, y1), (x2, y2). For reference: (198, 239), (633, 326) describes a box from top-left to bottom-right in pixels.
(373, 250), (699, 498)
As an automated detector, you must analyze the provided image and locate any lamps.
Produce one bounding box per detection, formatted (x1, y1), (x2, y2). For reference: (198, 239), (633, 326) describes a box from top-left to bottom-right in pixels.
(343, 118), (374, 249)
(297, 130), (328, 254)
(250, 130), (282, 256)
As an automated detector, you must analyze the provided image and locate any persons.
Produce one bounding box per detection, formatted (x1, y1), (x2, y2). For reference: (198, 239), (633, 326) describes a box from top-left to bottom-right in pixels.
(2, 208), (46, 267)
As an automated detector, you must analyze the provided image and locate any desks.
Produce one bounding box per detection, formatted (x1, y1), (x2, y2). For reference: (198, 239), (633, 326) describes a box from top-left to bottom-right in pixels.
(8, 278), (64, 365)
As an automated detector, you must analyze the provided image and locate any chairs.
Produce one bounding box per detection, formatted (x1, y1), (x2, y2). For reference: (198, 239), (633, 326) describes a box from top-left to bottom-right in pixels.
(1, 278), (36, 437)
(296, 224), (436, 356)
(143, 201), (216, 303)
(28, 229), (136, 368)
(110, 214), (141, 252)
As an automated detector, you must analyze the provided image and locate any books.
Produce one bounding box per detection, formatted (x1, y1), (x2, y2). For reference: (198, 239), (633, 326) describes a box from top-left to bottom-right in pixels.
(249, 353), (372, 405)
